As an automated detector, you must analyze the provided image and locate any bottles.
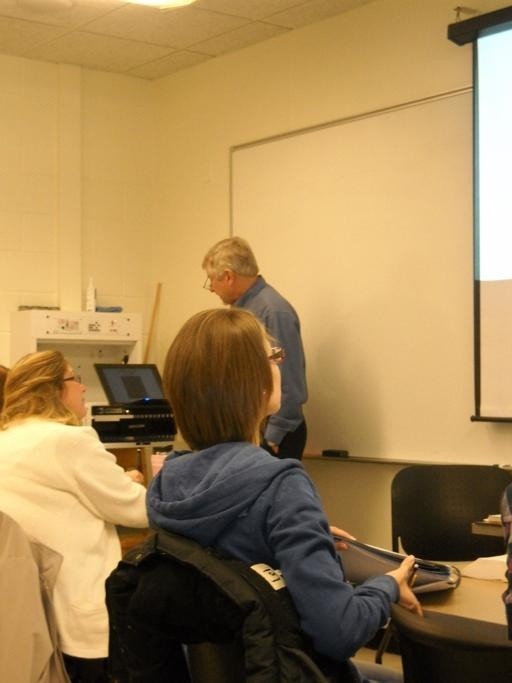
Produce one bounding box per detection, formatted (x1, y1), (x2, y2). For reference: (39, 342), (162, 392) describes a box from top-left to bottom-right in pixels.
(86, 277), (96, 312)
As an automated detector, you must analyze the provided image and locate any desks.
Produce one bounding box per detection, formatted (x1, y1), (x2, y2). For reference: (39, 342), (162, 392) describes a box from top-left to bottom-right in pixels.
(391, 560), (511, 681)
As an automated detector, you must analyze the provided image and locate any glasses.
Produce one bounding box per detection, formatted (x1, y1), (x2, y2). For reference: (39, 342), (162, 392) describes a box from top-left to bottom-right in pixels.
(203, 274), (214, 292)
(63, 374), (82, 384)
(266, 346), (286, 365)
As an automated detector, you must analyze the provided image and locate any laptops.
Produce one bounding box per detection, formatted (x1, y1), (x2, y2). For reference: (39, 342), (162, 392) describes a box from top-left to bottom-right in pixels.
(93, 363), (170, 406)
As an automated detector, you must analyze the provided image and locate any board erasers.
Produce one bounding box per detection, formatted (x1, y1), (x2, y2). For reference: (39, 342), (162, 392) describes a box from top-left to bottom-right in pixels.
(323, 450), (349, 459)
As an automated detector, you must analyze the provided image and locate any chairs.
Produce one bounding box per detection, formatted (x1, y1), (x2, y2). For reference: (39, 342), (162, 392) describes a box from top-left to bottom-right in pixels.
(374, 463), (511, 664)
(105, 557), (358, 683)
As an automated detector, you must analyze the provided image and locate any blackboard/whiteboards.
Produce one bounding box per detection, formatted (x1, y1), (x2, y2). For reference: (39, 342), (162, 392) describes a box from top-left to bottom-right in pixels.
(229, 86), (512, 475)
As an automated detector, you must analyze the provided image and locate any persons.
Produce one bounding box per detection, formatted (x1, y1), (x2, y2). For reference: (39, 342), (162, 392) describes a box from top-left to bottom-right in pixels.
(1, 348), (150, 682)
(142, 304), (426, 683)
(201, 235), (310, 462)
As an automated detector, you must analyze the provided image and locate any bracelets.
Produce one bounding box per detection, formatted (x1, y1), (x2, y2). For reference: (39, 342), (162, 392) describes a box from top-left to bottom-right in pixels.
(264, 439), (280, 448)
